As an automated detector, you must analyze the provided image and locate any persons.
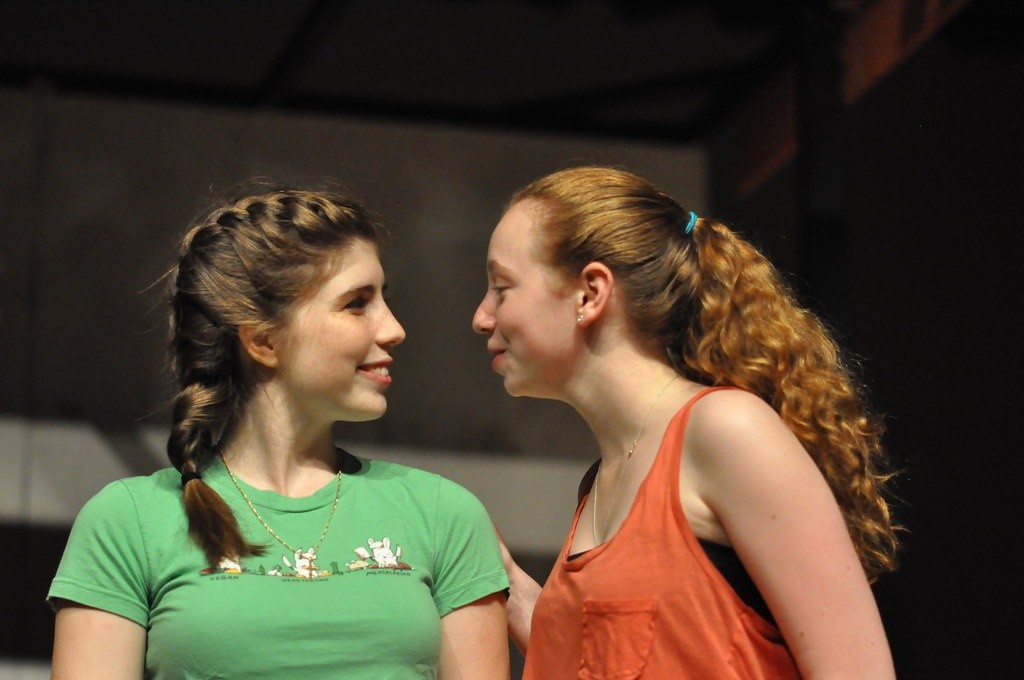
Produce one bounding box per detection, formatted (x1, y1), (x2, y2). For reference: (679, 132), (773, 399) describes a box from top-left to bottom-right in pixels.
(46, 190), (512, 680)
(472, 164), (901, 680)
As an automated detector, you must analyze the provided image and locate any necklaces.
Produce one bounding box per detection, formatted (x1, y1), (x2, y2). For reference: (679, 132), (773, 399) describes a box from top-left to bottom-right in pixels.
(588, 372), (683, 550)
(220, 442), (344, 582)
